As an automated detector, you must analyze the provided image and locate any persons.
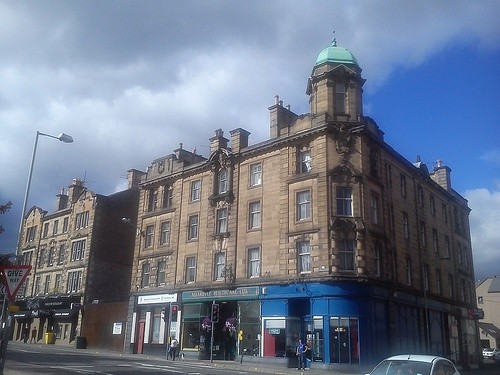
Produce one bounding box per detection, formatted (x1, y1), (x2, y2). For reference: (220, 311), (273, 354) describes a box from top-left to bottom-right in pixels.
(23, 325), (37, 344)
(169, 336), (178, 361)
(296, 338), (308, 371)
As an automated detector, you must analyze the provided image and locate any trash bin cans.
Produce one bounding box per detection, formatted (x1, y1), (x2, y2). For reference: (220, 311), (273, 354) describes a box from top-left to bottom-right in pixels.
(74, 336), (86, 349)
(45, 333), (54, 344)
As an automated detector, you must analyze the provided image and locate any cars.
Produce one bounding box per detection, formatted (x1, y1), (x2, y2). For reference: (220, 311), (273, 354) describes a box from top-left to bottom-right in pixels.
(364, 354), (461, 375)
(482, 348), (500, 359)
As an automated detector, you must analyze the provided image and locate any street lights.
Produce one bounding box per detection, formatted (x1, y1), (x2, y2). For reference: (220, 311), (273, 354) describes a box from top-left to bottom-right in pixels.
(0, 130), (74, 334)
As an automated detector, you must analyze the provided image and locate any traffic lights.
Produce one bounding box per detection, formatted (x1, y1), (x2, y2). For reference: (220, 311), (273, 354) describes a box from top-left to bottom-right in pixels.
(161, 308), (168, 323)
(212, 304), (219, 323)
(239, 330), (245, 341)
(171, 305), (178, 323)
